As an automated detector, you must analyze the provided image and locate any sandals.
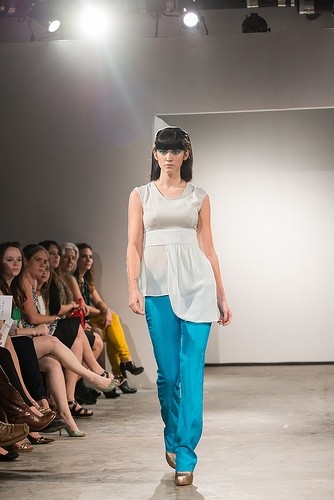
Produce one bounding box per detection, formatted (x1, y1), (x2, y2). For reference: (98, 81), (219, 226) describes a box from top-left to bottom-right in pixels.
(69, 400), (94, 418)
(100, 370), (123, 384)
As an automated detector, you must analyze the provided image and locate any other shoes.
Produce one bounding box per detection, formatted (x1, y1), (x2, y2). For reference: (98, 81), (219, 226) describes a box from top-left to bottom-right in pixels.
(0, 448), (19, 461)
(166, 451), (176, 469)
(26, 433), (56, 444)
(39, 419), (66, 433)
(0, 422), (29, 447)
(175, 472), (194, 486)
(74, 378), (100, 405)
(15, 442), (33, 452)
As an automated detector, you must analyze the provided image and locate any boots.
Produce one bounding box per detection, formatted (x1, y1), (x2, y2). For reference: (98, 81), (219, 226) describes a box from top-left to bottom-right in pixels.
(0, 366), (57, 431)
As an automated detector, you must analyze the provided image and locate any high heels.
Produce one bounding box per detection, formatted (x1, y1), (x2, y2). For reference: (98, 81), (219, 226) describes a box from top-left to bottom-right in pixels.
(113, 376), (138, 394)
(83, 377), (119, 394)
(56, 414), (86, 437)
(119, 361), (144, 378)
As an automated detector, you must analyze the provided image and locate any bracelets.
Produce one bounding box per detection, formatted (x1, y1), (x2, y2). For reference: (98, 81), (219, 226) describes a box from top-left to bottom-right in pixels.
(54, 315), (58, 320)
(103, 308), (110, 313)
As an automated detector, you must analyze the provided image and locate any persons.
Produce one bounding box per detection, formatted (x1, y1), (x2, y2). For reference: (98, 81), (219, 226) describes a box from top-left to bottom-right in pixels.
(0, 240), (145, 461)
(126, 126), (232, 485)
